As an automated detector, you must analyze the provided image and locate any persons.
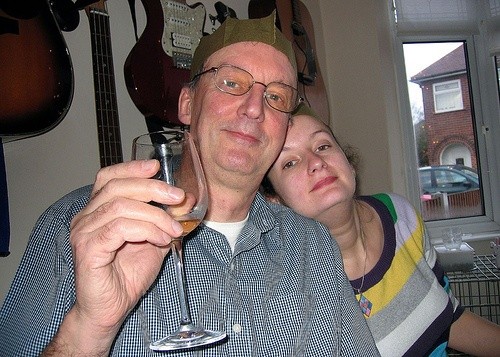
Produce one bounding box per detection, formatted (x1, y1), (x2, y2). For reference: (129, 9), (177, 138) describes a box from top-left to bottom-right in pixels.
(0, 9), (380, 356)
(261, 111), (500, 357)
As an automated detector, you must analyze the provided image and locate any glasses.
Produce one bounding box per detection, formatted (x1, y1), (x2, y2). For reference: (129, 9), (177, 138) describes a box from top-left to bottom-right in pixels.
(188, 63), (305, 115)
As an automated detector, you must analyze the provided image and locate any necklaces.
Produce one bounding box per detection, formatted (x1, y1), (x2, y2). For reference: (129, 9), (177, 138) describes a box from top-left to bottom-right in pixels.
(352, 234), (372, 317)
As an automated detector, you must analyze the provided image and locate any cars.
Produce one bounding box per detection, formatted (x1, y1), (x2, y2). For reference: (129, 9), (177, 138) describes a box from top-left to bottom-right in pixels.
(418, 164), (479, 196)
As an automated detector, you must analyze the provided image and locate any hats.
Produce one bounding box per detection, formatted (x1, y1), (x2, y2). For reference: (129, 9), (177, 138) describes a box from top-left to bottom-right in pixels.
(190, 9), (298, 85)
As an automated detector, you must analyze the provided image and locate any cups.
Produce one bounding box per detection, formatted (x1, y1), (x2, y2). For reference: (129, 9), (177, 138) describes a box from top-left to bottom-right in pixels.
(441, 227), (463, 250)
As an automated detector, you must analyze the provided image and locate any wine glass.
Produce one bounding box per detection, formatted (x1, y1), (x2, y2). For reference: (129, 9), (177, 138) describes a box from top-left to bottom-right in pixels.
(131, 130), (226, 351)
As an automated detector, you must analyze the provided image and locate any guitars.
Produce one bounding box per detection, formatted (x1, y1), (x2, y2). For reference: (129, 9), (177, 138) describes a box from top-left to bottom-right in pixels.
(80, 0), (124, 168)
(123, 0), (206, 126)
(247, 0), (331, 126)
(0, 0), (75, 142)
